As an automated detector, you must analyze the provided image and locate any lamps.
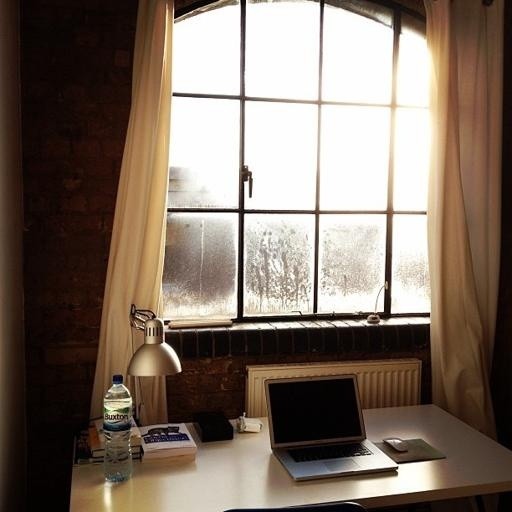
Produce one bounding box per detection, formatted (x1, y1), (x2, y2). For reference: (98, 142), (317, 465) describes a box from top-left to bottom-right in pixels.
(126, 302), (183, 427)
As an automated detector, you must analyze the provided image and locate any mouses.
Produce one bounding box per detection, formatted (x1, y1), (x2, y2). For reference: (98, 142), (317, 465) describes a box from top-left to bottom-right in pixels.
(383, 438), (409, 452)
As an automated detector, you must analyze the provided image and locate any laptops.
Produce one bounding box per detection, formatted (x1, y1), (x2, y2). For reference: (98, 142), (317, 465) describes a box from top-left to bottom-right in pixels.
(264, 374), (399, 481)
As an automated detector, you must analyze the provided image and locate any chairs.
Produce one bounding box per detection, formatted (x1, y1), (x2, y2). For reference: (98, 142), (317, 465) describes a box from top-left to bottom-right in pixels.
(223, 501), (369, 512)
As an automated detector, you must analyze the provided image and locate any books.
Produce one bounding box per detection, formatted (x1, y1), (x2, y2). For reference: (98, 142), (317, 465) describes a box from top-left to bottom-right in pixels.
(76, 416), (199, 465)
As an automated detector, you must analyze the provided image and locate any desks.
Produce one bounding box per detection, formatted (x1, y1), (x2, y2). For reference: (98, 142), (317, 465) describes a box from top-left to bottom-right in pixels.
(69, 403), (512, 512)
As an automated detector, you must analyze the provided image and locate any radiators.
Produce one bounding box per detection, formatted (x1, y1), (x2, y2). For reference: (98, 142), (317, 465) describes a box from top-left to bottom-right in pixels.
(245, 359), (423, 419)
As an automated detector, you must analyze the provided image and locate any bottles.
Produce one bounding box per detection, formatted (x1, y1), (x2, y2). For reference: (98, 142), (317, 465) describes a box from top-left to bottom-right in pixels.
(103, 375), (133, 483)
